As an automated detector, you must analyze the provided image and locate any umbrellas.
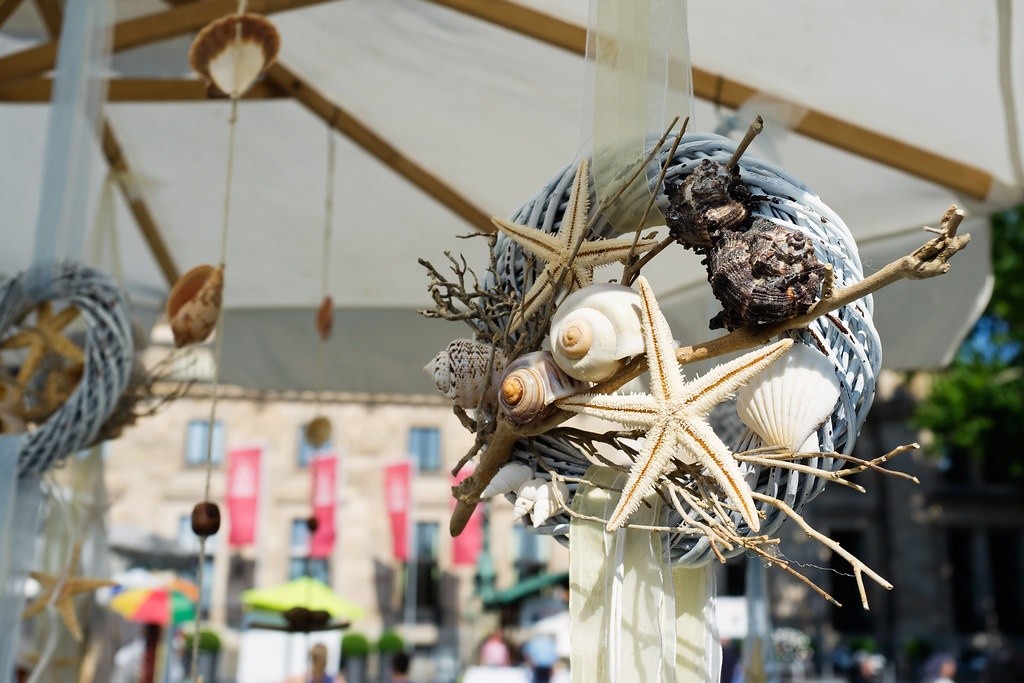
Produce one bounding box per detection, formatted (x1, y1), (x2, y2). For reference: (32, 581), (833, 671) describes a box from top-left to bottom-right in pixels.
(240, 574), (370, 672)
(96, 567), (204, 626)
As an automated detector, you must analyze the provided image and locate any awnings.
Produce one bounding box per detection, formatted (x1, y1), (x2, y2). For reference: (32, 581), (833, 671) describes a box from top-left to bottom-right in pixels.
(481, 571), (570, 613)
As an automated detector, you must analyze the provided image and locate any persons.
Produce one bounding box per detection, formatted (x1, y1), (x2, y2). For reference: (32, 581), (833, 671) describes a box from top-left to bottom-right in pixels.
(386, 651), (416, 683)
(781, 637), (958, 683)
(306, 642), (338, 683)
(517, 573), (572, 682)
